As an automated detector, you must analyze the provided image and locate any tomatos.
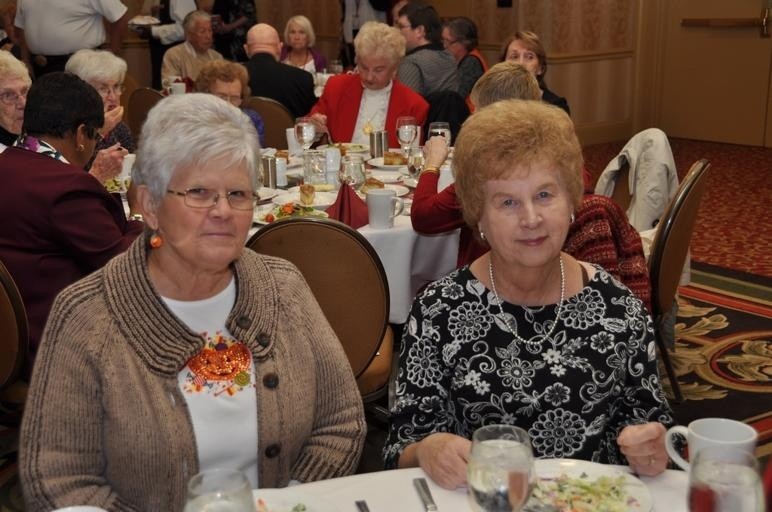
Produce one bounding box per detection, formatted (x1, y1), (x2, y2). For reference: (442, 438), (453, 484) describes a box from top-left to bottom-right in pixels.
(264, 213), (274, 222)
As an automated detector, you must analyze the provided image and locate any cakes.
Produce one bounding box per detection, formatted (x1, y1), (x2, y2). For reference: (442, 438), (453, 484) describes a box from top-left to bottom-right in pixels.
(275, 150), (289, 165)
(384, 151), (407, 165)
(300, 184), (315, 205)
(361, 178), (384, 195)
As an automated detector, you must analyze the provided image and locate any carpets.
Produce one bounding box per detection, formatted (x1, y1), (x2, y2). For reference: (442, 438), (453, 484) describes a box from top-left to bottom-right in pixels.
(582, 136), (772, 476)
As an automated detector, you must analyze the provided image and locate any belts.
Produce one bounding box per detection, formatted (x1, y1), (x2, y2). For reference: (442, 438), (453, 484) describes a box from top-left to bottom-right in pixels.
(28, 53), (73, 67)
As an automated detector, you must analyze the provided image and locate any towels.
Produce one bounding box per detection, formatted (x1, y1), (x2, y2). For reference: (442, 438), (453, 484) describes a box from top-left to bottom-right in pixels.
(325, 181), (370, 229)
(164, 75), (194, 96)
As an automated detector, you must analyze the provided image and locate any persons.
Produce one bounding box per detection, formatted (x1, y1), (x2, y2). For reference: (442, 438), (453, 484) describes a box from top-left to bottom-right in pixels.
(161, 13), (229, 99)
(130, 0), (199, 93)
(411, 62), (543, 271)
(382, 100), (679, 489)
(192, 62), (265, 148)
(0, 73), (136, 395)
(278, 16), (327, 77)
(17, 93), (366, 509)
(393, 5), (461, 119)
(239, 24), (318, 121)
(14, 0), (127, 75)
(503, 32), (570, 118)
(439, 17), (488, 116)
(0, 49), (35, 158)
(210, 0), (257, 63)
(63, 49), (135, 174)
(293, 22), (429, 148)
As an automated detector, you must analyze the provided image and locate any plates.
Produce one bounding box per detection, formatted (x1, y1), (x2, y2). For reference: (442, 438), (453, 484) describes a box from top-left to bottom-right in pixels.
(367, 156), (409, 170)
(364, 169), (410, 183)
(252, 202), (329, 225)
(403, 175), (421, 189)
(272, 192), (338, 210)
(316, 143), (370, 153)
(355, 183), (410, 200)
(520, 458), (653, 512)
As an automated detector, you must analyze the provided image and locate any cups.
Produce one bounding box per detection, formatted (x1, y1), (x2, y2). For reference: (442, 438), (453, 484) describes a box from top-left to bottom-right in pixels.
(365, 189), (404, 229)
(397, 116), (418, 160)
(665, 417), (758, 474)
(436, 165), (454, 194)
(687, 450), (768, 512)
(276, 158), (288, 186)
(467, 424), (535, 511)
(407, 146), (425, 184)
(184, 467), (254, 512)
(428, 122), (451, 149)
(303, 149), (328, 185)
(294, 117), (316, 151)
(286, 128), (304, 158)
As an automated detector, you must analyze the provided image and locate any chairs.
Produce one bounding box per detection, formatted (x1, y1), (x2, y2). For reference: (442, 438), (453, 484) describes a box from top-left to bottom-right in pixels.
(607, 128), (672, 225)
(242, 96), (293, 150)
(645, 158), (710, 403)
(126, 87), (165, 143)
(247, 218), (395, 409)
(1, 264), (32, 433)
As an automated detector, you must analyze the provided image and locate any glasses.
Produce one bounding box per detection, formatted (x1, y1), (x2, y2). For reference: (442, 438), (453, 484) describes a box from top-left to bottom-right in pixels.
(97, 84), (125, 97)
(400, 23), (414, 30)
(168, 186), (263, 209)
(219, 95), (243, 105)
(86, 129), (105, 142)
(1, 88), (29, 104)
(445, 38), (460, 45)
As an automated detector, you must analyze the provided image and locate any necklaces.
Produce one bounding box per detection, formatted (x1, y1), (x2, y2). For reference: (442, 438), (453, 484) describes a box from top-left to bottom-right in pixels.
(362, 87), (390, 134)
(490, 253), (564, 345)
